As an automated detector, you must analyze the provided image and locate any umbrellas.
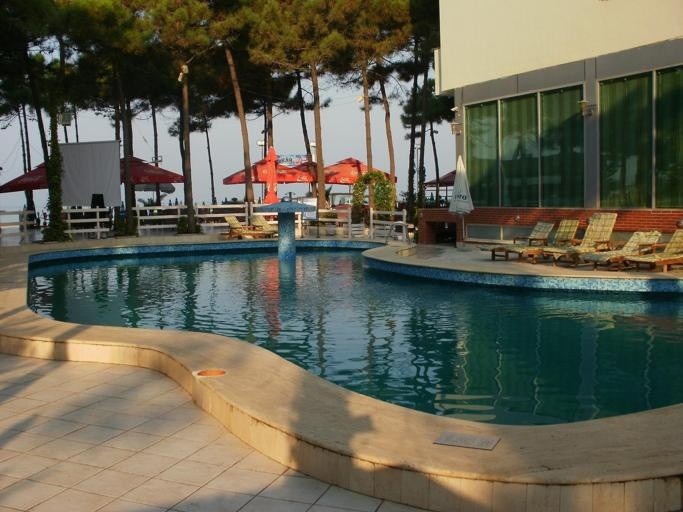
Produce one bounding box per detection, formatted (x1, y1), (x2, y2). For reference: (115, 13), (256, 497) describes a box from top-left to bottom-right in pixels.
(448, 155), (475, 240)
(223, 146), (397, 204)
(119, 156), (185, 207)
(0, 158), (49, 206)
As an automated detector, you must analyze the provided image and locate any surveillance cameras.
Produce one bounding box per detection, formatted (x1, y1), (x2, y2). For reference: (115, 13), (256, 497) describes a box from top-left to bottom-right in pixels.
(450, 106), (459, 111)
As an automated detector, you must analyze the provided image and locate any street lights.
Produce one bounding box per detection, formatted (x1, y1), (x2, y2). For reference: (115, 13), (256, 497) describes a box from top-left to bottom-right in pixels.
(414, 143), (420, 194)
(255, 140), (265, 201)
(310, 141), (317, 148)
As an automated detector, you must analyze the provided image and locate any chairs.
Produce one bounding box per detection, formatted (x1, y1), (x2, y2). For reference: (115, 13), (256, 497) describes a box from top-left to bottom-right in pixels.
(222, 214), (278, 241)
(475, 212), (683, 273)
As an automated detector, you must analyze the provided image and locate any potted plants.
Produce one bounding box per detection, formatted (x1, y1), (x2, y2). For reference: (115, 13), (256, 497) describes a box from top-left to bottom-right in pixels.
(320, 210), (337, 236)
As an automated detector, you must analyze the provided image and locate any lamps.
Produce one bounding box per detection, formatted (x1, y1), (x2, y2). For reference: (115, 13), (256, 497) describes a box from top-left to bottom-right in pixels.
(447, 119), (462, 135)
(577, 99), (594, 117)
